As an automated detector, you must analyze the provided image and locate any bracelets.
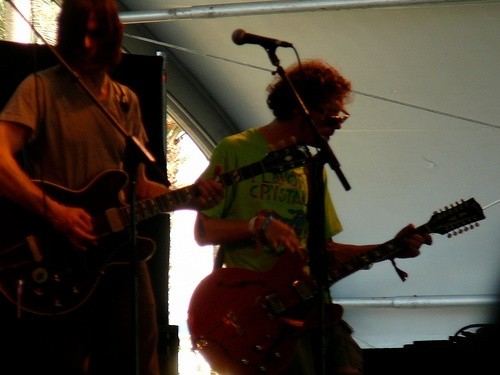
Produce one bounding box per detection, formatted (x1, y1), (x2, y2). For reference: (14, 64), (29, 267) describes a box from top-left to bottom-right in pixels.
(248, 215), (273, 239)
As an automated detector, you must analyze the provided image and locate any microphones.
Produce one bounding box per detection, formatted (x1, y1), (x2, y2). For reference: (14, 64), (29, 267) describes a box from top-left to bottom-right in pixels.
(231, 28), (294, 48)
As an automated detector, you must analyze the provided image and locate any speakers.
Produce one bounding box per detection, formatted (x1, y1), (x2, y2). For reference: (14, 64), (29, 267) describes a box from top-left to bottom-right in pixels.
(0, 40), (171, 375)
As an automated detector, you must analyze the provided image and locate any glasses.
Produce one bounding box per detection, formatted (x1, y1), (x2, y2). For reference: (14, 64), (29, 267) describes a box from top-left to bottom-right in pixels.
(323, 109), (349, 125)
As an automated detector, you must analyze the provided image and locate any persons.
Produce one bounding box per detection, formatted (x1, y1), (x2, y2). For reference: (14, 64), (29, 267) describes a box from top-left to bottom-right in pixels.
(0, 0), (223, 375)
(194, 58), (433, 375)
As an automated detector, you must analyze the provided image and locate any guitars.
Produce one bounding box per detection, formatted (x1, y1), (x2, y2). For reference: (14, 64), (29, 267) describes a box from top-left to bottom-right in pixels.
(0, 142), (320, 315)
(187, 196), (487, 375)
